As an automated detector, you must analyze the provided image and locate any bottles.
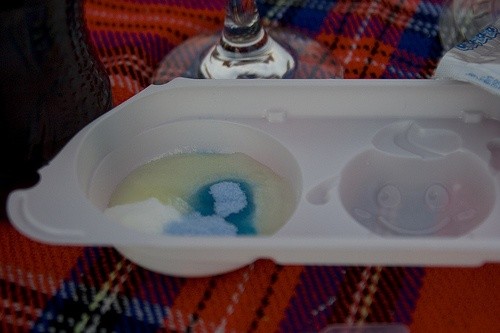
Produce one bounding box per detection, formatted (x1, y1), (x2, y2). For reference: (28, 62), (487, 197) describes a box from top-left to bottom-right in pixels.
(0, 0), (117, 218)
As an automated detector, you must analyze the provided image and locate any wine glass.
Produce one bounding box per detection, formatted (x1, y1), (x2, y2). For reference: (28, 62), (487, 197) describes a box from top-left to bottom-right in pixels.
(152, 0), (345, 84)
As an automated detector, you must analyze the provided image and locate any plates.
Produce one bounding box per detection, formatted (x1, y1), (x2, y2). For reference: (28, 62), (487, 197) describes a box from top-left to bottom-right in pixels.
(7, 75), (499, 275)
